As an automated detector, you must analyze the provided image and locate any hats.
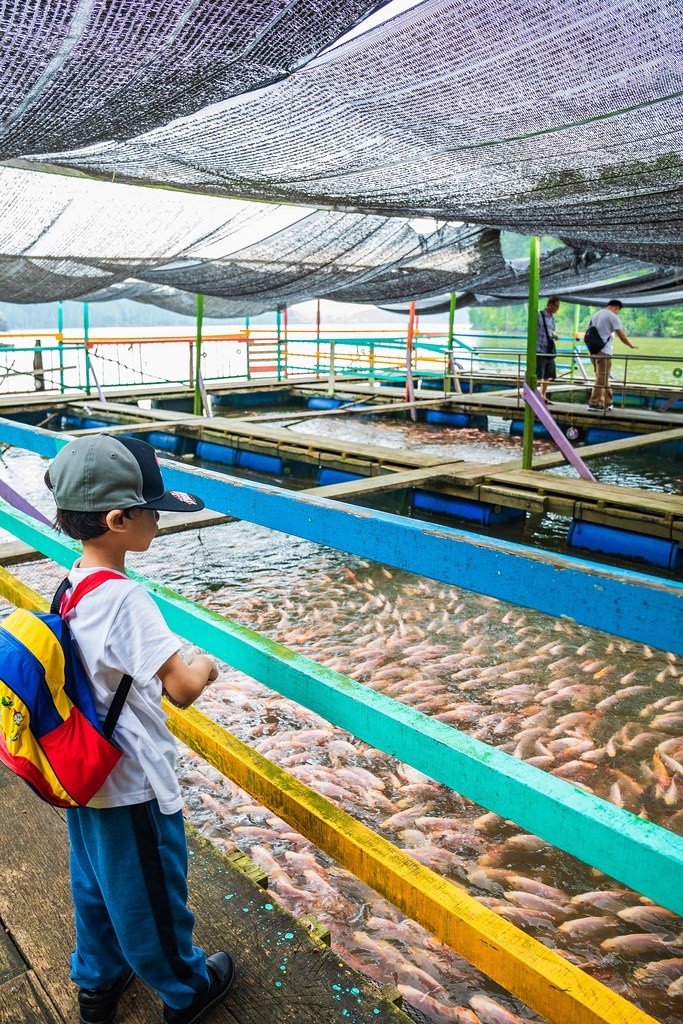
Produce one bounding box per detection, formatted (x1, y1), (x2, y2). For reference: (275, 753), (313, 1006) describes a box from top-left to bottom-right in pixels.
(47, 433), (204, 512)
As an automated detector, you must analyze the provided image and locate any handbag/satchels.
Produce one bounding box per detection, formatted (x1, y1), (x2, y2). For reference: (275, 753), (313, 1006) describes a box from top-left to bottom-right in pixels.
(585, 326), (605, 355)
(547, 336), (556, 358)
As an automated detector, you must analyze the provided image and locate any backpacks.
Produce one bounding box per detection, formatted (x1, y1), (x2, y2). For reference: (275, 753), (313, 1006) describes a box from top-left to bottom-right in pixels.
(1, 572), (132, 807)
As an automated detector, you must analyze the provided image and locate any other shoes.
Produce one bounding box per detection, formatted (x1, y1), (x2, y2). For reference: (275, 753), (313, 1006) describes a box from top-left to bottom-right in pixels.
(544, 400), (555, 406)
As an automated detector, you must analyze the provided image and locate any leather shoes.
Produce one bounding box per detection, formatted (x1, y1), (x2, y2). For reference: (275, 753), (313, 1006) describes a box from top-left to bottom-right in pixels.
(161, 950), (235, 1024)
(78, 966), (133, 1024)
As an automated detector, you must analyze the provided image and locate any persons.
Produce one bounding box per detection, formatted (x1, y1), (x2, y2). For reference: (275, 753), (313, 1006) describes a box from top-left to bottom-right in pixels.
(41, 433), (240, 1023)
(535, 295), (561, 406)
(586, 300), (639, 413)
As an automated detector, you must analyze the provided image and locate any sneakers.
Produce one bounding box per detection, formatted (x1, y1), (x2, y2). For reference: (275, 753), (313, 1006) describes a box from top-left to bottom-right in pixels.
(588, 404), (609, 411)
(606, 403), (614, 411)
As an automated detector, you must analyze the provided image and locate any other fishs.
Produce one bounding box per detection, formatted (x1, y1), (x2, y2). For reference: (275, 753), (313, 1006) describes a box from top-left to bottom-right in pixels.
(2, 553), (683, 1023)
